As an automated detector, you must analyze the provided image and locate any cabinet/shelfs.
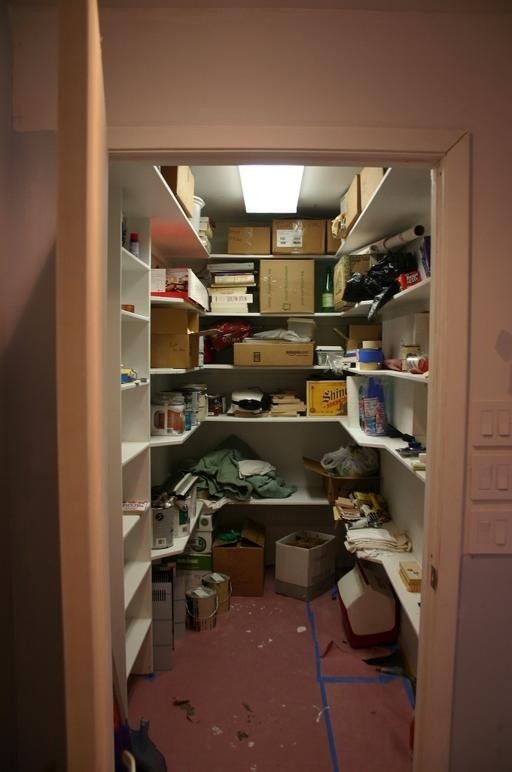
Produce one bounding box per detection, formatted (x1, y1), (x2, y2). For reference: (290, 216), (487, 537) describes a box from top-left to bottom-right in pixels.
(107, 152), (152, 702)
(335, 160), (433, 632)
(151, 165), (211, 562)
(212, 249), (334, 505)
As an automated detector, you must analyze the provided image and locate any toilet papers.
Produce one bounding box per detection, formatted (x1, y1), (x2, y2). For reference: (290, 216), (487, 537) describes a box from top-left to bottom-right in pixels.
(347, 376), (369, 426)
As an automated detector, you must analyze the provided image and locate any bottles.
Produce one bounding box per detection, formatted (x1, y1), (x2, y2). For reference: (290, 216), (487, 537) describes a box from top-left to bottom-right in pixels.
(130, 232), (141, 255)
(320, 267), (335, 312)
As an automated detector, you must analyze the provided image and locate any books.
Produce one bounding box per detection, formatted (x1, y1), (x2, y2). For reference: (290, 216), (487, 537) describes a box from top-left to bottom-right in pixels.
(207, 262), (257, 313)
(199, 217), (216, 253)
(269, 393), (307, 416)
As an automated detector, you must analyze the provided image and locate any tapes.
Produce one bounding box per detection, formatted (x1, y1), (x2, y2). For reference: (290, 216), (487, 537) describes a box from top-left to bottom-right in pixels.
(358, 341), (384, 370)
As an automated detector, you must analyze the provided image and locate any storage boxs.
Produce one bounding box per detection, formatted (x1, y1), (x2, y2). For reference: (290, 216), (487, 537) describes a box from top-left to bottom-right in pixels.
(227, 217), (335, 255)
(303, 454), (379, 501)
(152, 306), (221, 369)
(163, 165), (197, 217)
(229, 338), (314, 365)
(260, 259), (319, 316)
(207, 522), (333, 600)
(339, 164), (383, 235)
